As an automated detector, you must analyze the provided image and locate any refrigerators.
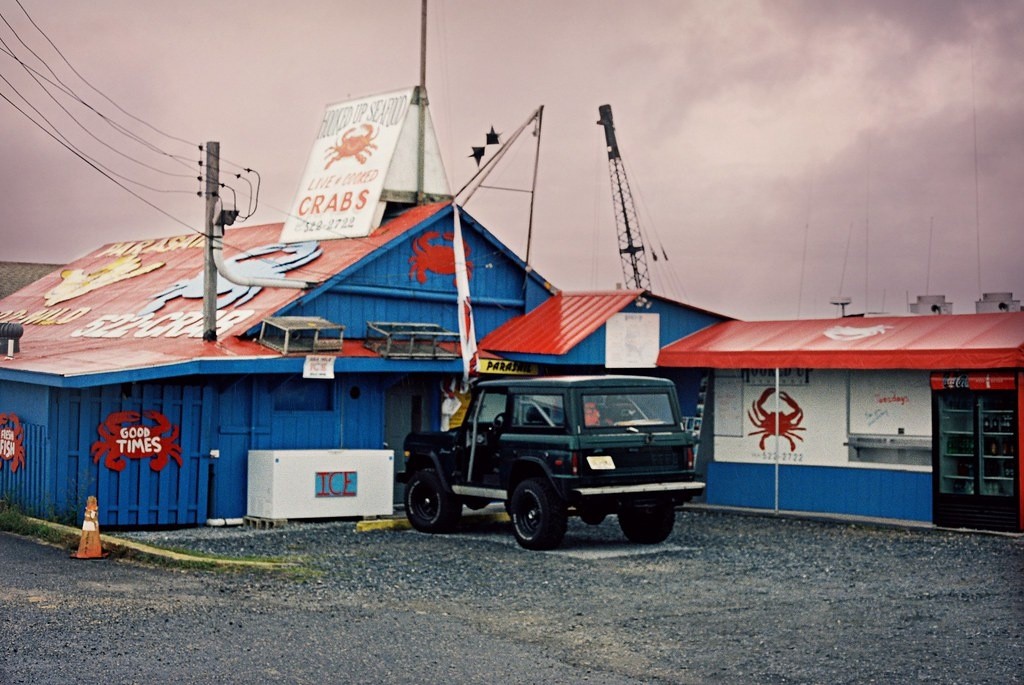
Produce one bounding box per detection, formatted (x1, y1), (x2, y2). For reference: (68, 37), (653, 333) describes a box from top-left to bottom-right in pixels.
(931, 371), (1024, 533)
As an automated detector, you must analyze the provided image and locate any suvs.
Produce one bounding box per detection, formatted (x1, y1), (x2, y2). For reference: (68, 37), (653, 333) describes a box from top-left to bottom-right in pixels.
(395, 373), (705, 553)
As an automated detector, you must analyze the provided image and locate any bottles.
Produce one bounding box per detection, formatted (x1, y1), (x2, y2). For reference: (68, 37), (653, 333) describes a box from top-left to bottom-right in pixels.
(983, 396), (1014, 496)
(946, 395), (975, 494)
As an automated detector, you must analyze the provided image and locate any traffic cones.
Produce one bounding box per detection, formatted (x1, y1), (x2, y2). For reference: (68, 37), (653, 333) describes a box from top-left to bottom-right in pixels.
(71, 494), (111, 560)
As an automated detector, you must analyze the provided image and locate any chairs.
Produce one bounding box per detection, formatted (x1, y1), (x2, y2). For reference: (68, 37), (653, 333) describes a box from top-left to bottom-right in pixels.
(521, 406), (551, 425)
(606, 403), (633, 422)
